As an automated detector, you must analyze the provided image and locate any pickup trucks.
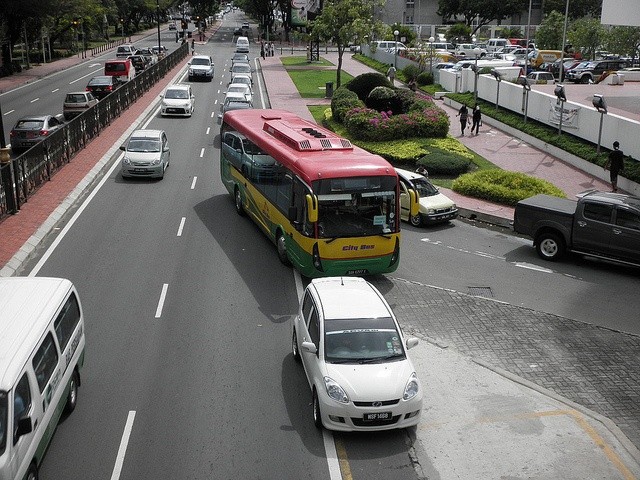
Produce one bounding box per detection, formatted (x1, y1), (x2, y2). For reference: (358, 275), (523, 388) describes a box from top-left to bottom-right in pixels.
(512, 191), (640, 273)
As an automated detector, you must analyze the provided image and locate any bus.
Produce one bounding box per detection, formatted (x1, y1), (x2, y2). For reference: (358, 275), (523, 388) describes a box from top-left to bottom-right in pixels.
(220, 107), (420, 281)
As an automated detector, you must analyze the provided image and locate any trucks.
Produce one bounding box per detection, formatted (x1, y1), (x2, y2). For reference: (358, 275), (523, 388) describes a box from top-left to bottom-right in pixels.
(451, 60), (524, 84)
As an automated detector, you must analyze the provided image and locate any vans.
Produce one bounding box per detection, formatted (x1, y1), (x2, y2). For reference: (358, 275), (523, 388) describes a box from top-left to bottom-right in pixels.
(367, 39), (408, 53)
(236, 36), (250, 52)
(104, 59), (136, 82)
(0, 276), (86, 480)
(532, 49), (562, 69)
(424, 43), (456, 52)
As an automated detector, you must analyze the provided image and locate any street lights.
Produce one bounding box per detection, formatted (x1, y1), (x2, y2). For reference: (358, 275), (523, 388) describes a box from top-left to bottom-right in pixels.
(428, 37), (435, 75)
(393, 30), (399, 71)
(491, 68), (502, 111)
(473, 47), (481, 100)
(519, 74), (530, 126)
(555, 83), (567, 136)
(592, 93), (607, 154)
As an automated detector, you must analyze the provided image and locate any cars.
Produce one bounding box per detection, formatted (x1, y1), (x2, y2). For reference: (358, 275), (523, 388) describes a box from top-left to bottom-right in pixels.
(219, 100), (252, 117)
(222, 131), (286, 183)
(188, 55), (214, 80)
(232, 53), (250, 66)
(433, 62), (455, 69)
(134, 48), (157, 64)
(152, 46), (168, 60)
(169, 23), (177, 30)
(567, 62), (591, 78)
(120, 129), (171, 180)
(292, 273), (421, 435)
(231, 63), (251, 80)
(559, 60), (580, 75)
(86, 75), (122, 99)
(160, 84), (195, 117)
(525, 71), (554, 85)
(125, 55), (149, 73)
(116, 45), (137, 58)
(612, 67), (640, 81)
(242, 22), (249, 29)
(487, 36), (531, 60)
(395, 167), (459, 227)
(226, 84), (252, 101)
(230, 76), (251, 90)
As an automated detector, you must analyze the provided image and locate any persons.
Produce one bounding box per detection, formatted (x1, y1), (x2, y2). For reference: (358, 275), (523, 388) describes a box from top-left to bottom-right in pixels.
(604, 141), (624, 192)
(387, 63), (396, 85)
(457, 105), (470, 136)
(417, 167), (426, 177)
(416, 164), (429, 179)
(470, 102), (482, 136)
(260, 41), (275, 60)
(408, 78), (417, 93)
(191, 39), (196, 55)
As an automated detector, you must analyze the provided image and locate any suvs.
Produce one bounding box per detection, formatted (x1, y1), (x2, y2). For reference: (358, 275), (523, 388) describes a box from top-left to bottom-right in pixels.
(536, 62), (565, 82)
(10, 114), (68, 155)
(64, 92), (99, 119)
(455, 44), (486, 59)
(540, 58), (576, 76)
(224, 92), (249, 104)
(569, 60), (630, 84)
